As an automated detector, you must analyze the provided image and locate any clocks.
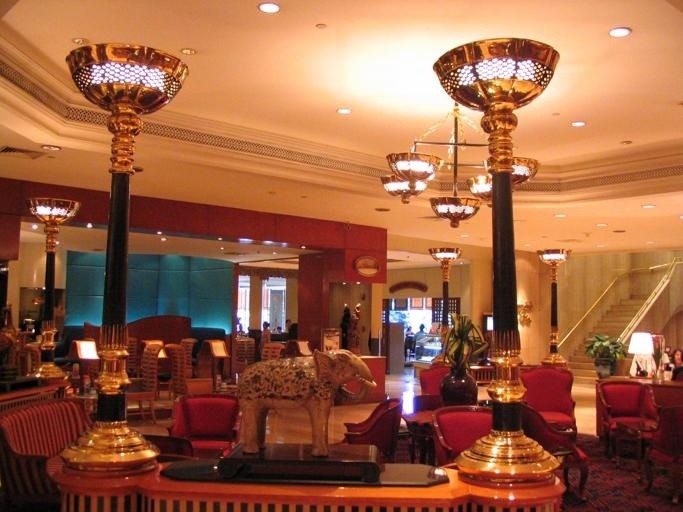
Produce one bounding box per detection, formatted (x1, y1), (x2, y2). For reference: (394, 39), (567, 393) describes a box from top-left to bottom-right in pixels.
(354, 256), (381, 278)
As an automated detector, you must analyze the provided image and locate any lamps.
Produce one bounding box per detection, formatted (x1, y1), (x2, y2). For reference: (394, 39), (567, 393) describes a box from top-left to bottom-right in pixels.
(433, 37), (560, 489)
(627, 333), (658, 378)
(27, 197), (81, 379)
(60, 43), (189, 478)
(536, 249), (572, 365)
(429, 247), (463, 365)
(381, 101), (541, 228)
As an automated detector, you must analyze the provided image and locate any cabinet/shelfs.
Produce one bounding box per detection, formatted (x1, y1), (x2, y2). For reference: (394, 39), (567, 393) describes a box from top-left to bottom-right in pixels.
(413, 333), (442, 383)
(382, 323), (404, 374)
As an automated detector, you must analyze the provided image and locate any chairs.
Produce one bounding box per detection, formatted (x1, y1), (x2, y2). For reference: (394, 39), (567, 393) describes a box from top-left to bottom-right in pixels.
(1, 326), (65, 384)
(420, 365), (683, 503)
(165, 344), (213, 400)
(124, 340), (164, 424)
(215, 329), (387, 395)
(180, 338), (197, 378)
(344, 398), (403, 462)
(166, 393), (243, 459)
(142, 434), (194, 463)
(126, 338), (137, 373)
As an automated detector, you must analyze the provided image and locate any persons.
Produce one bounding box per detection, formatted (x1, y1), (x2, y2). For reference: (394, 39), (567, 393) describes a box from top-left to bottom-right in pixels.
(413, 323), (426, 342)
(277, 326), (282, 334)
(260, 321), (270, 350)
(245, 327), (249, 335)
(238, 324), (243, 332)
(405, 326), (413, 335)
(664, 344), (683, 371)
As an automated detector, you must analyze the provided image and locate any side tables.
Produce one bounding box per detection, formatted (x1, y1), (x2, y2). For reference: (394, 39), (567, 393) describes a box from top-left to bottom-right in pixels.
(403, 411), (436, 465)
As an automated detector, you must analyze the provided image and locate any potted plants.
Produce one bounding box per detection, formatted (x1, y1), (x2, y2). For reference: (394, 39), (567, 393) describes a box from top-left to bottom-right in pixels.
(436, 313), (488, 406)
(585, 334), (627, 378)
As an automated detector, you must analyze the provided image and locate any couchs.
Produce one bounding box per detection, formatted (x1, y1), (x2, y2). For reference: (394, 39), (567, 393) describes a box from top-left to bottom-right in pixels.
(84, 316), (191, 376)
(1, 383), (142, 506)
